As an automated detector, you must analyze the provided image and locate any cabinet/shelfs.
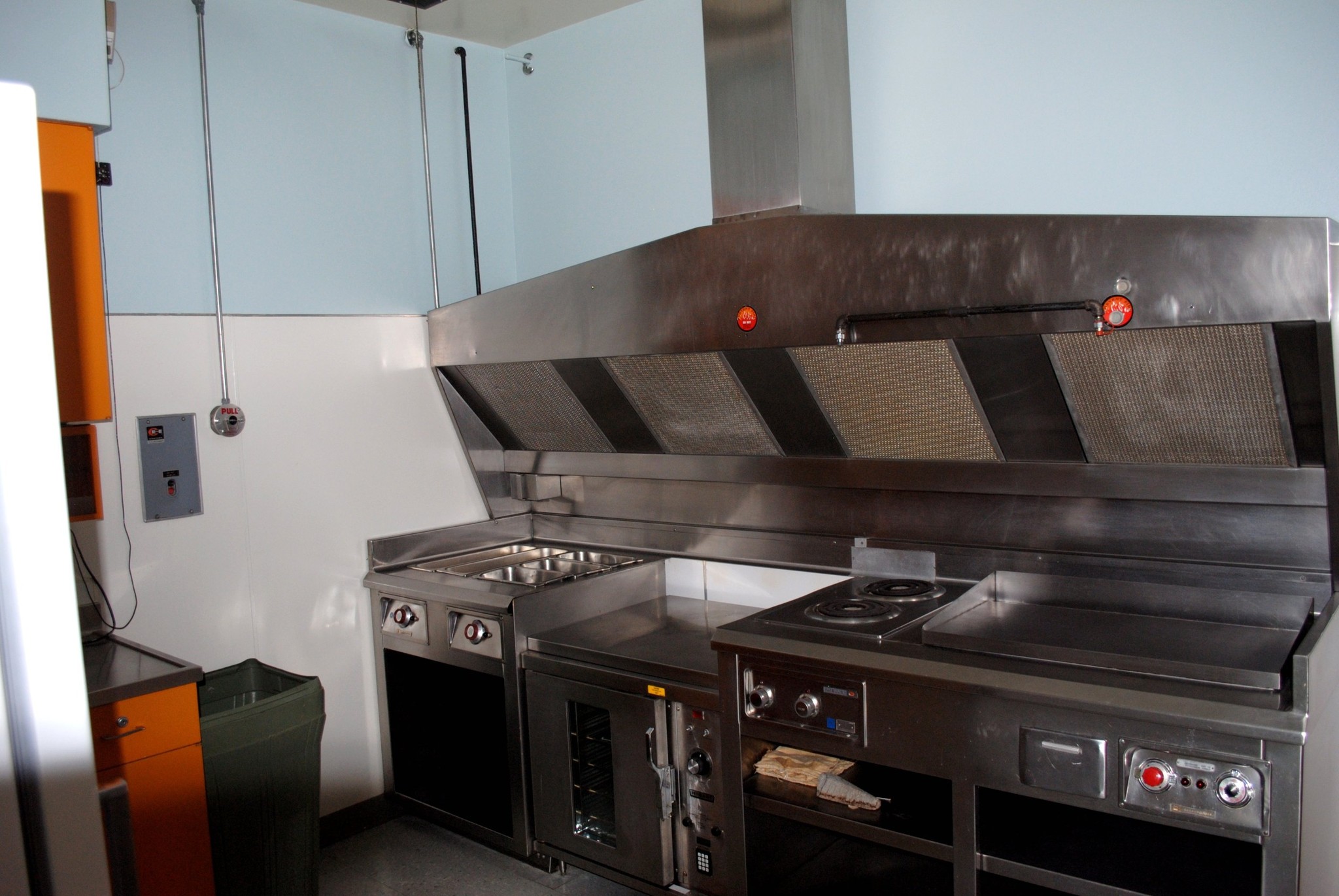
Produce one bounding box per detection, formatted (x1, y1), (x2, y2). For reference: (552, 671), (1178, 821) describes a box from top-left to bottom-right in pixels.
(78, 624), (216, 896)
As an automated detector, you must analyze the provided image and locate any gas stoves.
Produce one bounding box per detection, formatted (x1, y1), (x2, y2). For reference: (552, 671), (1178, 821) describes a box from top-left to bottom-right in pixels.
(748, 556), (977, 764)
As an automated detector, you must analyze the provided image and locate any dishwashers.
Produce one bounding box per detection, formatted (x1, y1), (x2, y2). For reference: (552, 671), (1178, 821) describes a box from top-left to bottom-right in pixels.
(523, 627), (751, 893)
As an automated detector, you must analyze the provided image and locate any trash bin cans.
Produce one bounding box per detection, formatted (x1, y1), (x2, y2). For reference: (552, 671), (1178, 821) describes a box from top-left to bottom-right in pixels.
(198, 658), (325, 896)
